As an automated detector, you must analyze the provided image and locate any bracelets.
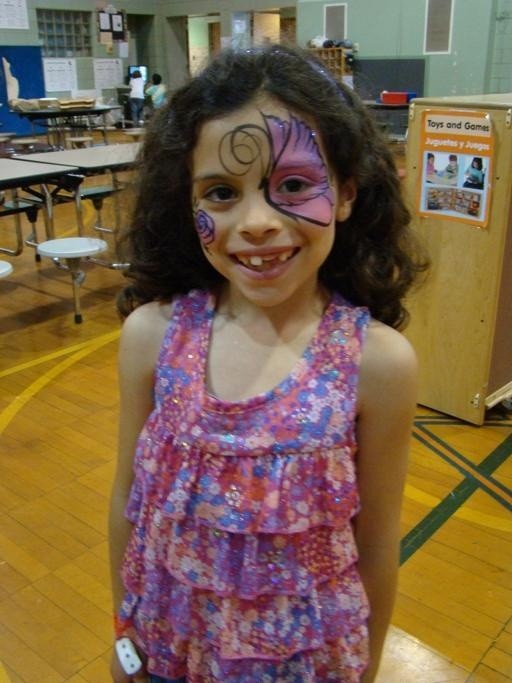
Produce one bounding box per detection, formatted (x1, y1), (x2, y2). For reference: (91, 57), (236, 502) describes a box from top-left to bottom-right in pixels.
(115, 637), (143, 675)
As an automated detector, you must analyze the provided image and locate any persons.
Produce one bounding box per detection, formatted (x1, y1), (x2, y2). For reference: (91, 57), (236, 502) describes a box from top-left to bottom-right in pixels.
(108, 37), (430, 682)
(128, 69), (146, 128)
(442, 153), (458, 179)
(144, 72), (169, 108)
(425, 152), (437, 177)
(462, 156), (484, 189)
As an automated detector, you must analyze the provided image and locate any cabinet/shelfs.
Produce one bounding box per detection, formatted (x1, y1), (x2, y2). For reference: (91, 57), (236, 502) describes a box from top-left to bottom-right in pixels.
(35, 7), (94, 58)
(306, 47), (353, 90)
(392, 91), (511, 427)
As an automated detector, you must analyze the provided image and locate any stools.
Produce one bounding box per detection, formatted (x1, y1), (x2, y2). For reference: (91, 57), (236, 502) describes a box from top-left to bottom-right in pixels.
(37, 237), (109, 322)
(0, 125), (146, 153)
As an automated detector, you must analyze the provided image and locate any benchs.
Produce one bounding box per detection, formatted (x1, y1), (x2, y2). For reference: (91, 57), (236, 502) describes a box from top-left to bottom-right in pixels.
(1, 182), (130, 261)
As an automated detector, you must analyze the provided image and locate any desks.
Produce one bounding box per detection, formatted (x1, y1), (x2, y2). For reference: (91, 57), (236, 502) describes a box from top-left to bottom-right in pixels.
(361, 98), (408, 134)
(11, 102), (122, 151)
(1, 139), (143, 268)
